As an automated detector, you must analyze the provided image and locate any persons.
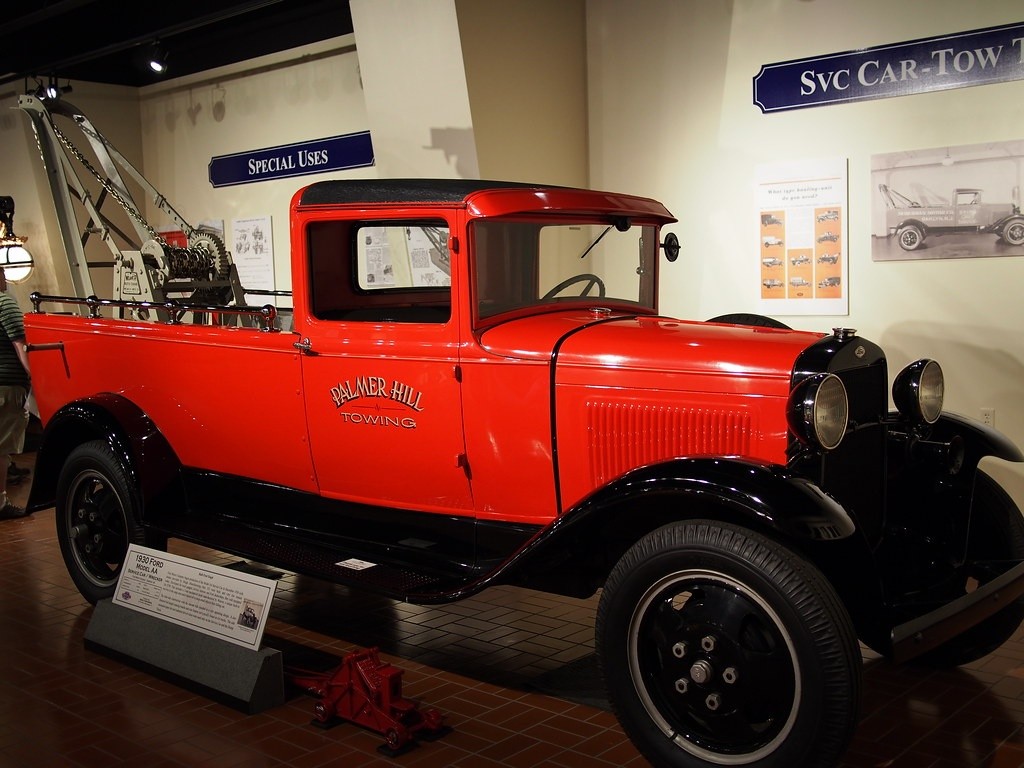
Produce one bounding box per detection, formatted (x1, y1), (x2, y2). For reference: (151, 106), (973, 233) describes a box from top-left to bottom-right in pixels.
(0, 267), (33, 523)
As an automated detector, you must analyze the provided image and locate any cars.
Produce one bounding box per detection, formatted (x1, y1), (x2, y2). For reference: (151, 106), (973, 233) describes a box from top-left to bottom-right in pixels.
(20, 175), (1024, 767)
(878, 181), (1024, 252)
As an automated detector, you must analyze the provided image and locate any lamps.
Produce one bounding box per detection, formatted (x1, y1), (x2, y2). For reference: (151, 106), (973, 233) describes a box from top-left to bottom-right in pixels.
(0, 195), (35, 285)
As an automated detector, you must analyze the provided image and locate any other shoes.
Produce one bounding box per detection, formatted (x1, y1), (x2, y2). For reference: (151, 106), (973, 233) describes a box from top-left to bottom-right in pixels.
(0, 497), (28, 519)
(7, 461), (32, 479)
